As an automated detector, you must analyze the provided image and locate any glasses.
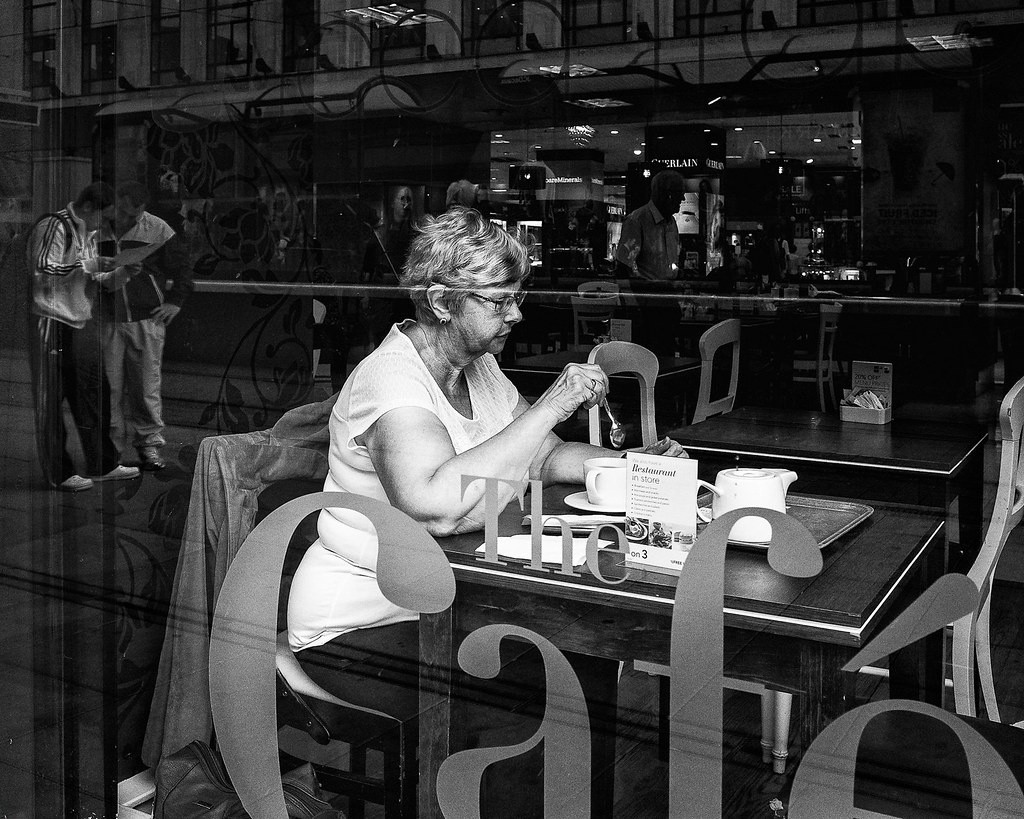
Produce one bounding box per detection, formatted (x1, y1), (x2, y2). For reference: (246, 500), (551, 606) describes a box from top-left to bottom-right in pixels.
(432, 280), (528, 315)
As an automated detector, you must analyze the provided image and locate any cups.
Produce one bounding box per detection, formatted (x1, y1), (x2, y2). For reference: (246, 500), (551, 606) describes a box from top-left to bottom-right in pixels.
(583, 458), (626, 505)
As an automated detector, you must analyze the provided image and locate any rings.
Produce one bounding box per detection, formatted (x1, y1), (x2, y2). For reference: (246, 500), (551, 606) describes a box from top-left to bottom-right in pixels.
(591, 377), (596, 390)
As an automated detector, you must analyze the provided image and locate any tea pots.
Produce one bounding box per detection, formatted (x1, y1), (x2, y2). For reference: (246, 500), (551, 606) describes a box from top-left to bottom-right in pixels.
(697, 468), (797, 544)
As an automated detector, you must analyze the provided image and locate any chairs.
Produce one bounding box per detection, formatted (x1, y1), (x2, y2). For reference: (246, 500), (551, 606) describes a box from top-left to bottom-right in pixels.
(118, 280), (1023, 818)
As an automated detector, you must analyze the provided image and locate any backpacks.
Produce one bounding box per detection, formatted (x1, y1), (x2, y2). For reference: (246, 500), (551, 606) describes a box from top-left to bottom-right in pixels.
(0, 210), (73, 318)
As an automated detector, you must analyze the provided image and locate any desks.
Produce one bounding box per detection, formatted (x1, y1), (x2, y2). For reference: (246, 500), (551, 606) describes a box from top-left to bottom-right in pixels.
(675, 312), (786, 402)
(518, 348), (701, 430)
(416, 488), (946, 818)
(658, 406), (991, 709)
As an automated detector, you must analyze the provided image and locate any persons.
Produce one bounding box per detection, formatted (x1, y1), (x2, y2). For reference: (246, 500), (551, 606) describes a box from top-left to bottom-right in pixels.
(52, 169), (875, 360)
(24, 183), (141, 496)
(359, 184), (415, 356)
(287, 206), (688, 819)
(101, 183), (195, 468)
(600, 168), (686, 450)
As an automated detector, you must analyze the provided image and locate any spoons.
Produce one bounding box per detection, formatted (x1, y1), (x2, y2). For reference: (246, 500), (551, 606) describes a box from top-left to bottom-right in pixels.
(604, 397), (626, 448)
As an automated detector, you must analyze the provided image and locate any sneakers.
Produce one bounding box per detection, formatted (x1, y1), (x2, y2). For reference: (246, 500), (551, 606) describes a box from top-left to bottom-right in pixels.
(91, 465), (140, 482)
(138, 449), (167, 471)
(46, 475), (94, 492)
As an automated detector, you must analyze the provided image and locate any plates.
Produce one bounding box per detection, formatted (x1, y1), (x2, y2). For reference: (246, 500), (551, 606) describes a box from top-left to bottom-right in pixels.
(564, 492), (626, 512)
